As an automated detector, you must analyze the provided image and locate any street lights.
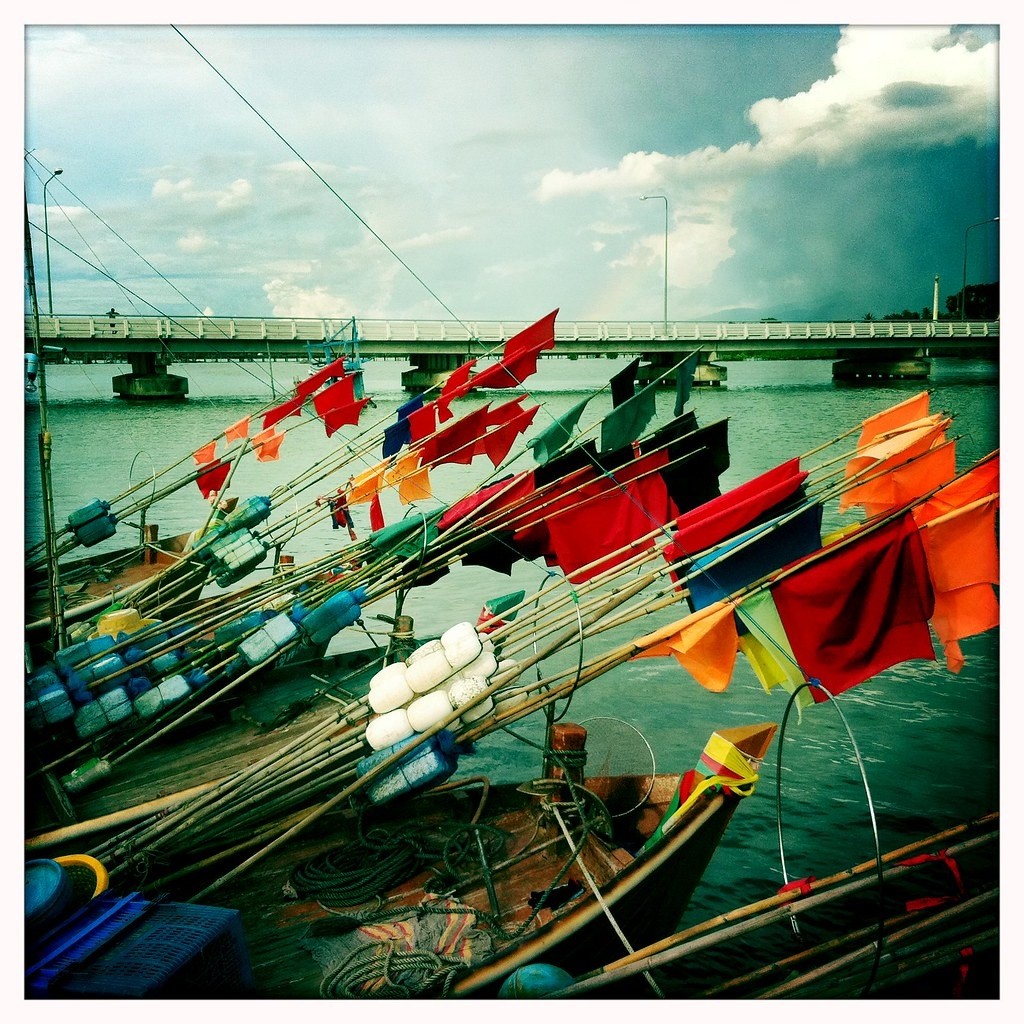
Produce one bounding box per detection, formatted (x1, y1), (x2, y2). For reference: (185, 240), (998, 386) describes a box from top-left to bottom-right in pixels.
(961, 217), (999, 324)
(638, 195), (670, 335)
(43, 168), (66, 319)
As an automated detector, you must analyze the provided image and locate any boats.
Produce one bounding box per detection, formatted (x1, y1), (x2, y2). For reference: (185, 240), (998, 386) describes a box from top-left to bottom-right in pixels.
(24, 190), (1000, 999)
